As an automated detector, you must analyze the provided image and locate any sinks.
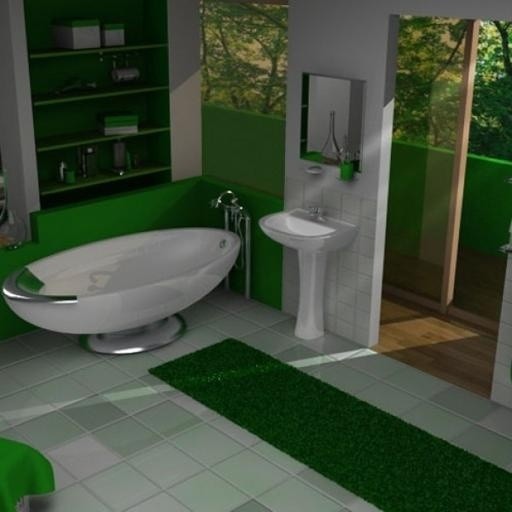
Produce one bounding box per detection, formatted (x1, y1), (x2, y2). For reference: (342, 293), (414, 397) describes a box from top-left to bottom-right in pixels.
(258, 207), (357, 252)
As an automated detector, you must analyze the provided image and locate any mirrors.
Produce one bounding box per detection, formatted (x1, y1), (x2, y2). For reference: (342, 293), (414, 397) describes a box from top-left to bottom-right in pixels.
(301, 72), (367, 176)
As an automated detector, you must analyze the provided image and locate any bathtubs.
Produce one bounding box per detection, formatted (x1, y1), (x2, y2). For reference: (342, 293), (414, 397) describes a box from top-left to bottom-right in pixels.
(0, 227), (243, 336)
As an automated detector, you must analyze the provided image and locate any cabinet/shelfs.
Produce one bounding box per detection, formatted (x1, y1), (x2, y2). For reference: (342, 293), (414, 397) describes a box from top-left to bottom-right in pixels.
(1, 0), (201, 242)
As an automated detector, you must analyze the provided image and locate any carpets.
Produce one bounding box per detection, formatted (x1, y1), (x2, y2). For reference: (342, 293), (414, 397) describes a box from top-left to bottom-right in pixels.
(147, 336), (511, 512)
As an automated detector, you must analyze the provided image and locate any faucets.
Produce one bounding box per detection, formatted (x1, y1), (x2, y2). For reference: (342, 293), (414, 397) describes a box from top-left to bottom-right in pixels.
(211, 189), (235, 209)
(308, 204), (325, 218)
(80, 146), (97, 179)
